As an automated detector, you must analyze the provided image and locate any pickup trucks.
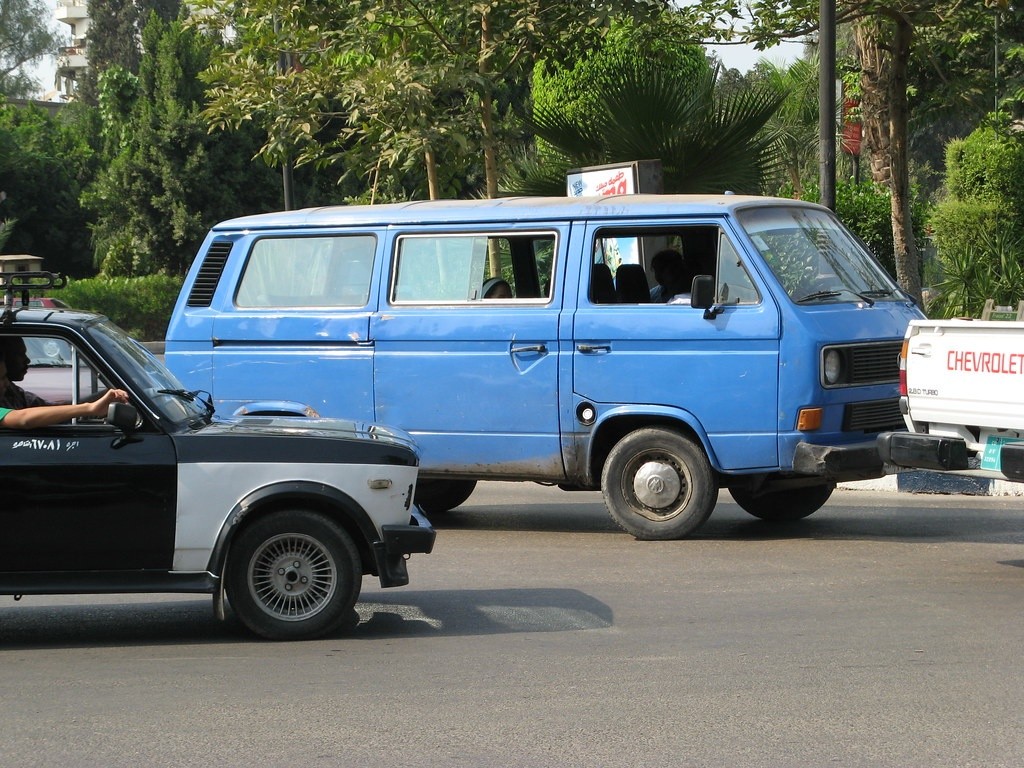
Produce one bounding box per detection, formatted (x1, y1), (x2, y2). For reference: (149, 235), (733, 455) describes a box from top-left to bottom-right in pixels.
(876, 300), (1024, 496)
(0, 271), (437, 640)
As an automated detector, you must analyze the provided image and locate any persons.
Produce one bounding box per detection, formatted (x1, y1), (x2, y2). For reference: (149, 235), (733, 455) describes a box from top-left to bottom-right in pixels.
(482, 278), (512, 298)
(0, 336), (130, 429)
(649, 249), (685, 303)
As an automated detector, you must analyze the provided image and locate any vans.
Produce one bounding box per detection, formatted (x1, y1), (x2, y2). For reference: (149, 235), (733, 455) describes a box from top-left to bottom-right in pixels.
(163, 194), (927, 540)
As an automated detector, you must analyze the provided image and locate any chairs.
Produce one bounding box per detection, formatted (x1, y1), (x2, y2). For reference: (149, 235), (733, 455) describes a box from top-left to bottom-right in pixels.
(616, 264), (650, 302)
(589, 264), (616, 305)
